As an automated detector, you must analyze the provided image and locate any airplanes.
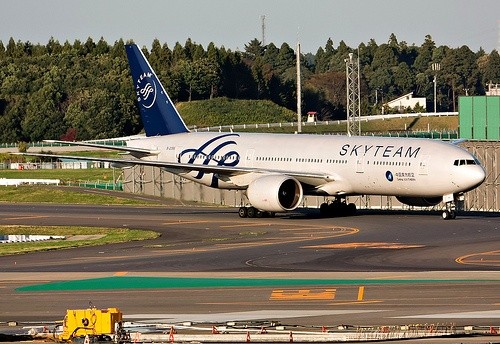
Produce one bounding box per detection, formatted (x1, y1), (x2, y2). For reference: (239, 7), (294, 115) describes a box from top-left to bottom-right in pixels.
(8, 44), (487, 220)
